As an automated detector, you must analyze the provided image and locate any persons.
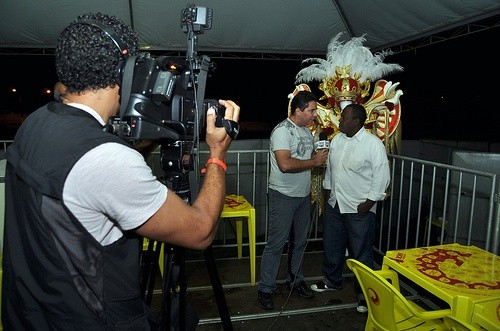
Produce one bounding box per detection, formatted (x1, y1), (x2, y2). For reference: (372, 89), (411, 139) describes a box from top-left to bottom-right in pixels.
(310, 104), (391, 313)
(255, 90), (328, 310)
(0, 12), (241, 331)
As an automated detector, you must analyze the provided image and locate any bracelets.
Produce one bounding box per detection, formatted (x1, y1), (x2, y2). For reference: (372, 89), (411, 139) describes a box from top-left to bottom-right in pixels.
(201, 158), (227, 173)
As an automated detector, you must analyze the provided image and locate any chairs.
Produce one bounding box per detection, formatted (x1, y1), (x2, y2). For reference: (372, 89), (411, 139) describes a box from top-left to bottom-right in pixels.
(347, 259), (450, 331)
(142, 237), (164, 280)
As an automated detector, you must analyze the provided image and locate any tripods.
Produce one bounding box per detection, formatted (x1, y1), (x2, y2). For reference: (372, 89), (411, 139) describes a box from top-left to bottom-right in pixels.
(140, 141), (234, 331)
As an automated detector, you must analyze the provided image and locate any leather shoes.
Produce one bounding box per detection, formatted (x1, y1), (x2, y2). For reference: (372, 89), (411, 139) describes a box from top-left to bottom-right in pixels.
(287, 279), (314, 298)
(257, 291), (274, 309)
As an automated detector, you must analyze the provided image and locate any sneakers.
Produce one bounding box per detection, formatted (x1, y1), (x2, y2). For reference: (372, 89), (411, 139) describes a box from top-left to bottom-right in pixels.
(311, 281), (340, 292)
(357, 299), (368, 312)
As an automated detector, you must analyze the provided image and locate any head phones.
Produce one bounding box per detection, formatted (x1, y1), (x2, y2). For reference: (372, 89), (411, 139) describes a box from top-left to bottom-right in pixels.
(61, 19), (131, 87)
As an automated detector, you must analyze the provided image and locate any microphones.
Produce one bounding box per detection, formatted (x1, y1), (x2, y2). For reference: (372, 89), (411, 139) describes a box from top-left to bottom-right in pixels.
(317, 133), (330, 151)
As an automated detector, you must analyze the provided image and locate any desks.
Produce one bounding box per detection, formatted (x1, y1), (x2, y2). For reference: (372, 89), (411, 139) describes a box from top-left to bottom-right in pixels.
(220, 195), (255, 285)
(422, 217), (448, 246)
(382, 244), (500, 331)
